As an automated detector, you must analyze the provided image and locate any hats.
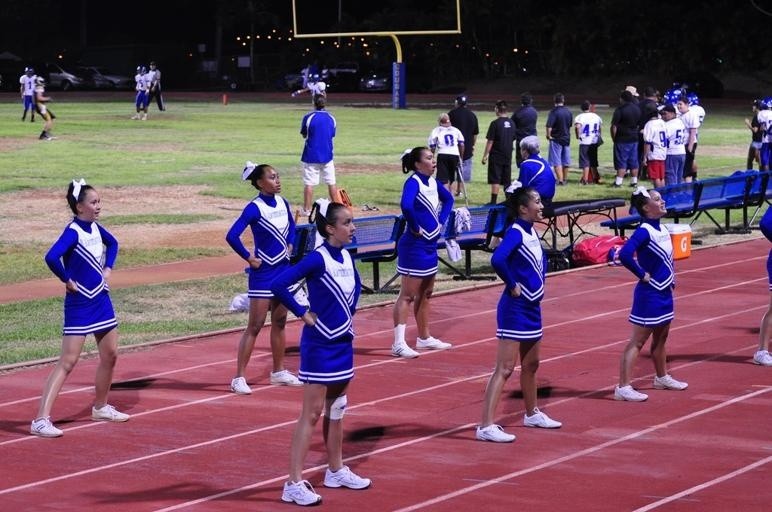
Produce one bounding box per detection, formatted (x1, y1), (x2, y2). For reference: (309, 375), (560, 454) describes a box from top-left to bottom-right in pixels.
(457, 95), (467, 105)
(621, 85), (640, 97)
(436, 113), (451, 127)
(150, 61), (157, 67)
(749, 99), (761, 107)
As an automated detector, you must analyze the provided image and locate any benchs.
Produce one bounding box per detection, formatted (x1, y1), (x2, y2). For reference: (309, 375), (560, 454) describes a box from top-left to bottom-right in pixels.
(600, 172), (769, 244)
(534, 199), (625, 248)
(291, 215), (399, 292)
(399, 207), (498, 280)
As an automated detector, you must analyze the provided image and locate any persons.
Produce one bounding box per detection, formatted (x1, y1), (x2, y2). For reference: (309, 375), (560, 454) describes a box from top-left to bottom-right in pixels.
(430, 113), (465, 196)
(614, 186), (689, 402)
(476, 180), (563, 443)
(20, 69), (37, 122)
(757, 98), (771, 171)
(744, 99), (763, 171)
(270, 197), (372, 506)
(753, 202), (772, 367)
(545, 93), (573, 184)
(30, 178), (132, 438)
(481, 100), (515, 206)
(300, 63), (329, 88)
(510, 92), (538, 168)
(291, 74), (327, 104)
(226, 159), (305, 395)
(610, 86), (704, 188)
(300, 95), (337, 217)
(447, 95), (479, 200)
(140, 62), (165, 111)
(32, 76), (56, 140)
(573, 100), (603, 185)
(391, 147), (452, 359)
(132, 66), (151, 121)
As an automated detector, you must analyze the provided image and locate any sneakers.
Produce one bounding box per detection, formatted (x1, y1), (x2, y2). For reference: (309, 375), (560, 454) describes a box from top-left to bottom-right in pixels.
(323, 464), (373, 490)
(523, 407), (562, 428)
(391, 341), (420, 359)
(39, 131), (59, 142)
(141, 114), (148, 121)
(580, 177), (588, 186)
(476, 422), (516, 445)
(555, 179), (568, 186)
(279, 478), (323, 508)
(626, 182), (637, 188)
(653, 374), (689, 391)
(593, 179), (599, 184)
(90, 404), (131, 422)
(605, 181), (623, 188)
(751, 350), (772, 366)
(270, 370), (304, 385)
(613, 384), (648, 402)
(30, 417), (65, 438)
(132, 115), (141, 121)
(230, 377), (252, 395)
(415, 335), (453, 350)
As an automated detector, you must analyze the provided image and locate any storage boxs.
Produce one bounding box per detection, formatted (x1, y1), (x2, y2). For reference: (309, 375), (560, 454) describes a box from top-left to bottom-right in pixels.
(665, 224), (692, 259)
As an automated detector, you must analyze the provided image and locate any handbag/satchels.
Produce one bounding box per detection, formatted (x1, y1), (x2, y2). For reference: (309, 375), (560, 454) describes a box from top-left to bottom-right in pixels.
(543, 246), (572, 272)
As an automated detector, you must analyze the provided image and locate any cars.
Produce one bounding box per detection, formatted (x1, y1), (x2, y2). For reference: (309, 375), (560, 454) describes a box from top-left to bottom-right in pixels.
(78, 62), (133, 90)
(358, 72), (389, 92)
(47, 64), (88, 90)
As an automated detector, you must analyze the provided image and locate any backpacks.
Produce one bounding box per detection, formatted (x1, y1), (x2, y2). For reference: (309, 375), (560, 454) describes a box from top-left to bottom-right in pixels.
(573, 234), (624, 265)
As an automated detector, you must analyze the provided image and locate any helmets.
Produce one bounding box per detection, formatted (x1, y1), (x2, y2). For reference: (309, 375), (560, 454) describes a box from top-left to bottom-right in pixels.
(24, 66), (35, 74)
(308, 73), (320, 83)
(687, 92), (701, 104)
(136, 65), (147, 75)
(661, 87), (683, 105)
(760, 96), (772, 109)
(34, 76), (44, 85)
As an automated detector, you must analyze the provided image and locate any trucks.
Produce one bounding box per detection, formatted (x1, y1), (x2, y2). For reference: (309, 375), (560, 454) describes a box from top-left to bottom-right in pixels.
(319, 66), (360, 81)
